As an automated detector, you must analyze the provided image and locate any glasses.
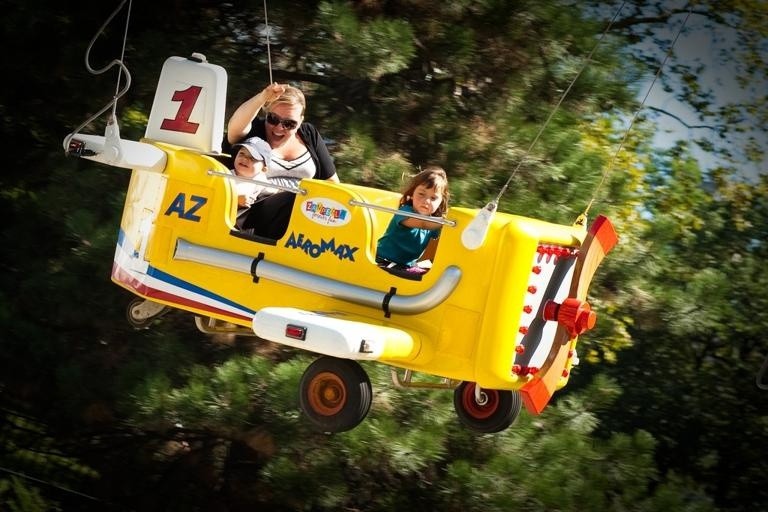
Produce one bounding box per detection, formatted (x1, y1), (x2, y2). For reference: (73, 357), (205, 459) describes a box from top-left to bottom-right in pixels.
(265, 110), (299, 131)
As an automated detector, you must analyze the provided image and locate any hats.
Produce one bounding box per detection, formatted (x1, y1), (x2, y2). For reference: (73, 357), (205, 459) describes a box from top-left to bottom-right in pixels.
(229, 135), (273, 171)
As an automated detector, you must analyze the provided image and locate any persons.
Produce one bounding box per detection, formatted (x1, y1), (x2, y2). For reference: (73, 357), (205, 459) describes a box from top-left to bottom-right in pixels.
(372, 164), (452, 281)
(228, 134), (287, 228)
(218, 80), (341, 243)
